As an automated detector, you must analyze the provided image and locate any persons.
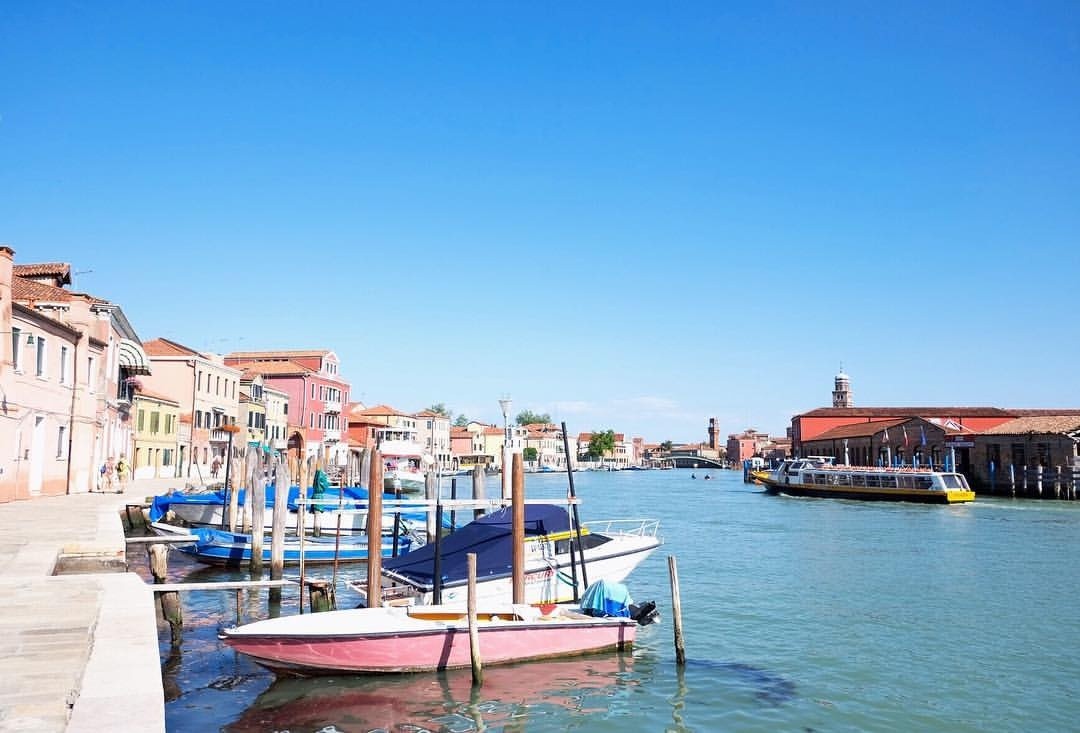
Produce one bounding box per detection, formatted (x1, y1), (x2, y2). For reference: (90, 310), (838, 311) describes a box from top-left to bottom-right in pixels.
(100, 452), (133, 494)
(212, 456), (222, 478)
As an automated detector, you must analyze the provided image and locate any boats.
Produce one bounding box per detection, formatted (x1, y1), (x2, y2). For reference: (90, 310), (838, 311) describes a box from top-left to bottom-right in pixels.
(216, 601), (638, 673)
(524, 467), (673, 472)
(749, 455), (975, 504)
(140, 468), (460, 568)
(345, 518), (663, 605)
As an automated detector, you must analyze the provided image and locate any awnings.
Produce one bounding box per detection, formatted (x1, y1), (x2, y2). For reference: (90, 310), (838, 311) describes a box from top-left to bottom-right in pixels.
(120, 337), (153, 376)
